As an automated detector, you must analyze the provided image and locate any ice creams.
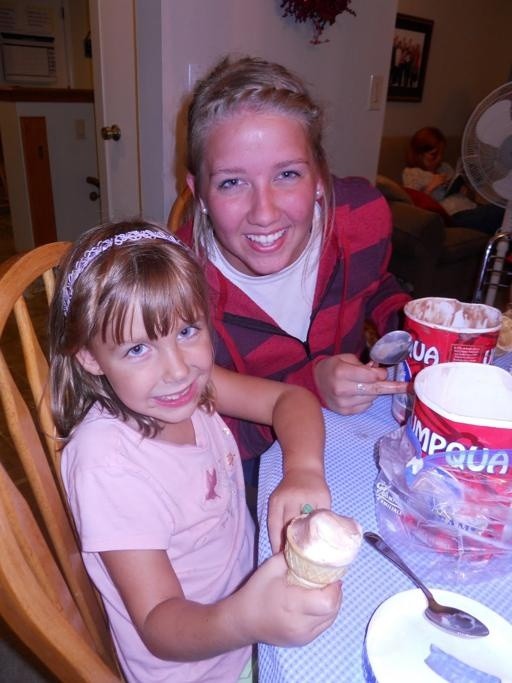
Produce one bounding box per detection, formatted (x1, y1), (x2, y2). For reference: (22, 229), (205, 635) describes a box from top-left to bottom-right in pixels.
(284, 508), (363, 589)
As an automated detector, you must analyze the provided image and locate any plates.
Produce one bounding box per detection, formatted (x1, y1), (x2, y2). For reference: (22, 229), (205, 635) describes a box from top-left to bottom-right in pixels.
(360, 588), (511, 683)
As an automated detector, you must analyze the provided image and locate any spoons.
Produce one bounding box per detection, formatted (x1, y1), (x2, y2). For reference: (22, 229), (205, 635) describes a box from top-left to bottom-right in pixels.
(361, 529), (491, 639)
(366, 330), (414, 367)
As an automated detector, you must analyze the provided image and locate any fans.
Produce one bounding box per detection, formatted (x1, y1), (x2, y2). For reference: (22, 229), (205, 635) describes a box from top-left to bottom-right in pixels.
(461, 82), (512, 307)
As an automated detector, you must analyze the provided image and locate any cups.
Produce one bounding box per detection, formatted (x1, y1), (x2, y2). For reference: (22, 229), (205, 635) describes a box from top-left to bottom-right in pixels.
(389, 294), (504, 424)
(395, 362), (511, 561)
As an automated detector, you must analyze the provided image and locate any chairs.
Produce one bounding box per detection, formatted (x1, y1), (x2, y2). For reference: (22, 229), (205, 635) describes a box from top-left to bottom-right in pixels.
(168, 185), (193, 234)
(377, 137), (508, 302)
(0, 241), (127, 683)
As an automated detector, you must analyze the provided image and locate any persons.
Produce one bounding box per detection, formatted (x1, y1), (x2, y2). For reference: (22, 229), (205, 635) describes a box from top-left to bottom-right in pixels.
(43, 215), (348, 683)
(402, 125), (501, 232)
(454, 142), (503, 210)
(388, 30), (422, 91)
(162, 53), (424, 482)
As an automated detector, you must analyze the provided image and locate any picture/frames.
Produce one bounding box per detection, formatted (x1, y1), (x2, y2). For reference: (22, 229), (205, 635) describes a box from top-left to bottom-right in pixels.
(387, 12), (434, 103)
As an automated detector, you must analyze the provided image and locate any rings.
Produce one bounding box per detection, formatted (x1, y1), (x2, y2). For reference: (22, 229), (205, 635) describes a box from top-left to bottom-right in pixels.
(354, 381), (366, 395)
(299, 503), (315, 513)
